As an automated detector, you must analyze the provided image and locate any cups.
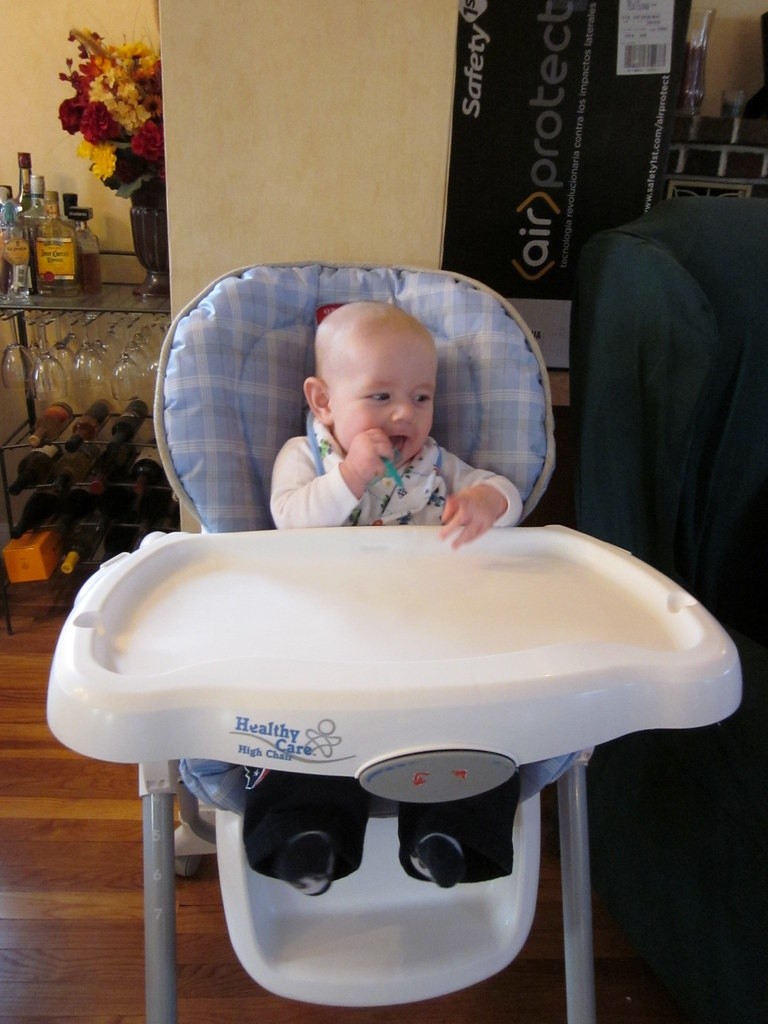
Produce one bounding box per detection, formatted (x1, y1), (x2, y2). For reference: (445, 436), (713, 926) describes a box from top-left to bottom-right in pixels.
(676, 6), (716, 116)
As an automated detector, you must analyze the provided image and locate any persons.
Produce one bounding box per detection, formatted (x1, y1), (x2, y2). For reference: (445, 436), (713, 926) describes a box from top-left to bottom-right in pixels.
(270, 302), (524, 549)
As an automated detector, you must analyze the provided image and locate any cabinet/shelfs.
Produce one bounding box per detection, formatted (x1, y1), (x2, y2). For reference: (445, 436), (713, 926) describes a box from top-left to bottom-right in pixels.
(0, 248), (180, 635)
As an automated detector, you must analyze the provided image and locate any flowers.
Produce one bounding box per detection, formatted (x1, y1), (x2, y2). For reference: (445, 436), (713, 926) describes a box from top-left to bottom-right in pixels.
(57, 9), (164, 199)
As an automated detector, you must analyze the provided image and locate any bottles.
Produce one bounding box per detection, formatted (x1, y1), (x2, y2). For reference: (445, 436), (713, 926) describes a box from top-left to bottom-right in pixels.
(0, 152), (101, 302)
(8, 399), (180, 573)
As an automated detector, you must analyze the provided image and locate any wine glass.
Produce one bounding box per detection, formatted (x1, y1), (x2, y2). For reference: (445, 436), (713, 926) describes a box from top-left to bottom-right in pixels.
(1, 306), (171, 402)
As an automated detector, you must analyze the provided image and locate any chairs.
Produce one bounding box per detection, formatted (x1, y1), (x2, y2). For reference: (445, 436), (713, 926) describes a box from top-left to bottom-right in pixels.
(47, 262), (743, 1024)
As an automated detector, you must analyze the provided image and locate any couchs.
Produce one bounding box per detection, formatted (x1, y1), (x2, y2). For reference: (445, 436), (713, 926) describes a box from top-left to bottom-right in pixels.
(570, 196), (768, 1024)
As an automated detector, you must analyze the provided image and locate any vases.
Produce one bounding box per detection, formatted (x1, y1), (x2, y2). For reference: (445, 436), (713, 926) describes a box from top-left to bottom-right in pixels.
(128, 178), (170, 299)
